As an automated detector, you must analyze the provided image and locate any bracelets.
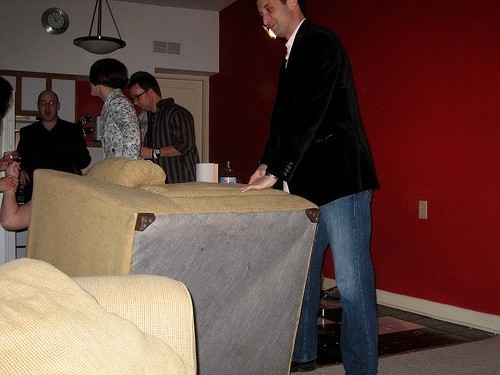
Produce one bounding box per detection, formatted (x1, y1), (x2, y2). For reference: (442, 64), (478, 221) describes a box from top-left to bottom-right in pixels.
(265, 173), (278, 180)
(152, 148), (154, 157)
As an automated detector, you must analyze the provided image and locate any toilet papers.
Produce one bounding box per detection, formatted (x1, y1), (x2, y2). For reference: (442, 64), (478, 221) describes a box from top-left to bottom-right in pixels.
(196, 163), (219, 183)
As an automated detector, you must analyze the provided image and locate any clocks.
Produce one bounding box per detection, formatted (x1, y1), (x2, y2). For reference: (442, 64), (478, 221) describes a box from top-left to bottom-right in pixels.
(41, 7), (70, 35)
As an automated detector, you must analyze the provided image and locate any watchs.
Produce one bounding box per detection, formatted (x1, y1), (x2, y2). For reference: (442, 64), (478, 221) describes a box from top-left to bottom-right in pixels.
(155, 148), (161, 159)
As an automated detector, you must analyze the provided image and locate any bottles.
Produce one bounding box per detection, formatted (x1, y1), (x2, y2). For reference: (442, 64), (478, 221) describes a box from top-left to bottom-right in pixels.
(220, 161), (238, 184)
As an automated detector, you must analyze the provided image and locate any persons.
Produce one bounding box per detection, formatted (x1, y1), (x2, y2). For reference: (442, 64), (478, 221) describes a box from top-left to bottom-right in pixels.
(240, 0), (381, 375)
(15, 90), (92, 203)
(89, 58), (141, 160)
(128, 71), (200, 184)
(1, 162), (31, 231)
(0, 77), (21, 192)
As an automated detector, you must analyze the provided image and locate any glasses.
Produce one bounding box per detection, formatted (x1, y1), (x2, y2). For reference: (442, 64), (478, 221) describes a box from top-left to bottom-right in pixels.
(130, 88), (150, 103)
(38, 101), (58, 107)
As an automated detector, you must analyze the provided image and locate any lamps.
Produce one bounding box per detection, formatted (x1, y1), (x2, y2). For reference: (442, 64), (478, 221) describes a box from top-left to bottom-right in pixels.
(72, 0), (126, 54)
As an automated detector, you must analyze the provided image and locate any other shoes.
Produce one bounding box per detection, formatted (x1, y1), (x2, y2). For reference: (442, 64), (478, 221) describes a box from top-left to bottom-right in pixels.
(290, 359), (317, 372)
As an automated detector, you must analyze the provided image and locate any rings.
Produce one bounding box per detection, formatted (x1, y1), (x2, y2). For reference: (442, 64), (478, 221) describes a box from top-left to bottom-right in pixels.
(13, 184), (15, 187)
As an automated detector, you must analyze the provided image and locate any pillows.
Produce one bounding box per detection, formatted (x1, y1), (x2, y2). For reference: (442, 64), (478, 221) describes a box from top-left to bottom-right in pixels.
(0, 256), (187, 375)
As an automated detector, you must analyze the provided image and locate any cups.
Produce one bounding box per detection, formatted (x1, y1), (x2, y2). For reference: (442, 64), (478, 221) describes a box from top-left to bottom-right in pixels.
(4, 151), (19, 166)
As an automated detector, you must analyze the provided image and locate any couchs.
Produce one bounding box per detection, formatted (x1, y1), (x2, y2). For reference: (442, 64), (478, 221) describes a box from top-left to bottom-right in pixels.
(24, 155), (319, 375)
